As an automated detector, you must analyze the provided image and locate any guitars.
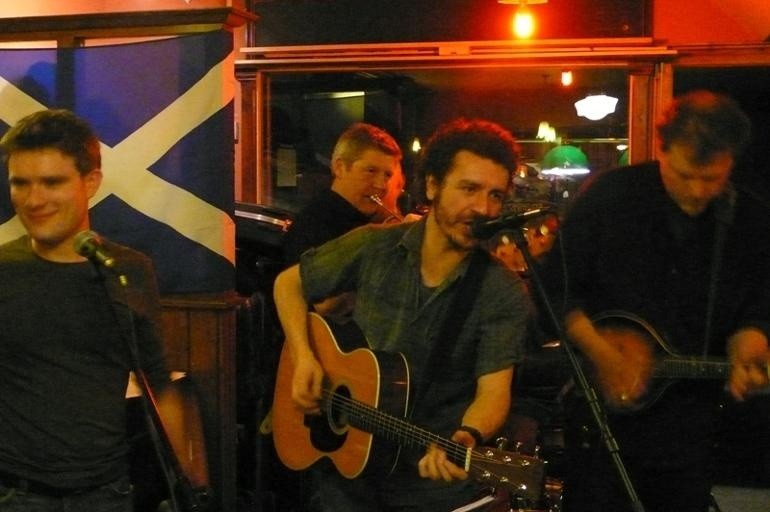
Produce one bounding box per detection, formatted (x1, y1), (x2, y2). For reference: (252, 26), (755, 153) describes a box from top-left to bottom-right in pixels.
(272, 311), (565, 511)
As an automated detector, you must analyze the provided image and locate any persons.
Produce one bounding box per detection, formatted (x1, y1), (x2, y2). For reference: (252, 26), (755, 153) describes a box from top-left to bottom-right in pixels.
(268, 120), (404, 327)
(528, 90), (768, 512)
(0, 109), (197, 511)
(275, 117), (533, 511)
(373, 154), (410, 225)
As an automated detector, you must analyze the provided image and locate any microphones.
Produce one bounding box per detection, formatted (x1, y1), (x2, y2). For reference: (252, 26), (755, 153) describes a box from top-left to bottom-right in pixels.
(73, 230), (122, 276)
(470, 206), (545, 240)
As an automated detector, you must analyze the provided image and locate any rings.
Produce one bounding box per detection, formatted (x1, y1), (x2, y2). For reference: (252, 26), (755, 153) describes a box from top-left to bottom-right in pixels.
(615, 390), (632, 402)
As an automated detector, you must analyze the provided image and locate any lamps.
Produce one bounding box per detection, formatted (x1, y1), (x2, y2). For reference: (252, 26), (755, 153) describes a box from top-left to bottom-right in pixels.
(539, 143), (592, 176)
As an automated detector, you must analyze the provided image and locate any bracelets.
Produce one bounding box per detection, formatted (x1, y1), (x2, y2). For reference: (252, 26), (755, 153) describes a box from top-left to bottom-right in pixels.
(456, 426), (483, 450)
